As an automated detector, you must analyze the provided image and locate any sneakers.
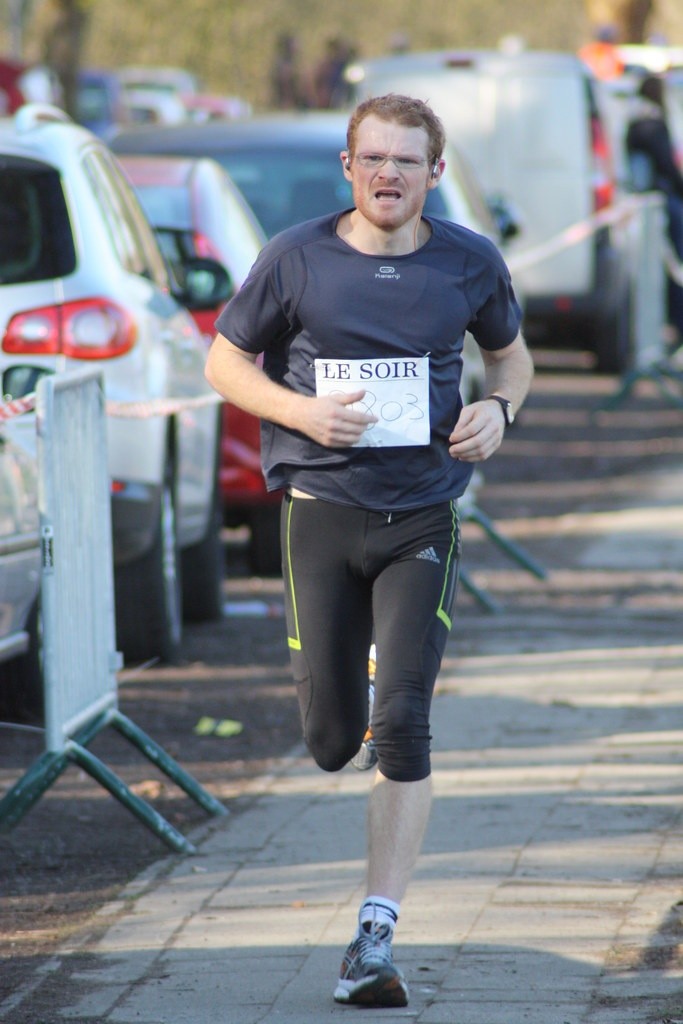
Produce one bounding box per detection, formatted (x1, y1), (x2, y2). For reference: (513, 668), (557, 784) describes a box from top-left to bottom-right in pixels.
(343, 642), (379, 772)
(333, 900), (411, 1007)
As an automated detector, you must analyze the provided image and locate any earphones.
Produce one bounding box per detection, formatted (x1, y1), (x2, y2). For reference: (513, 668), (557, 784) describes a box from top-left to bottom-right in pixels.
(345, 155), (350, 171)
(431, 158), (439, 179)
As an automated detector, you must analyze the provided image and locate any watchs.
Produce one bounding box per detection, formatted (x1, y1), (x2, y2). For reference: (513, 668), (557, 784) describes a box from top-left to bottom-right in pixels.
(486, 395), (514, 428)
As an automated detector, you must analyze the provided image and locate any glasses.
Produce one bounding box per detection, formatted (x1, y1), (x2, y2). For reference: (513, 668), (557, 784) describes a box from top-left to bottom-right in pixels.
(345, 152), (427, 169)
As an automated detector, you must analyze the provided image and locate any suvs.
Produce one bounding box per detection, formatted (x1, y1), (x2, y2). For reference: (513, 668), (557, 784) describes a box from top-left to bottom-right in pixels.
(0, 99), (240, 662)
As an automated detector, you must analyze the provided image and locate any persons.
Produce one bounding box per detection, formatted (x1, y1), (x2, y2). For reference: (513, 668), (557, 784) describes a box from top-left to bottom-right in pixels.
(204, 94), (534, 1008)
(262, 29), (352, 110)
(44, 0), (89, 122)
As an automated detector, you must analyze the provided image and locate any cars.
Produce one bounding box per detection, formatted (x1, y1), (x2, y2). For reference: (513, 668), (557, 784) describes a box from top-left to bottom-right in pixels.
(105, 147), (292, 584)
(2, 34), (683, 389)
(0, 352), (61, 691)
